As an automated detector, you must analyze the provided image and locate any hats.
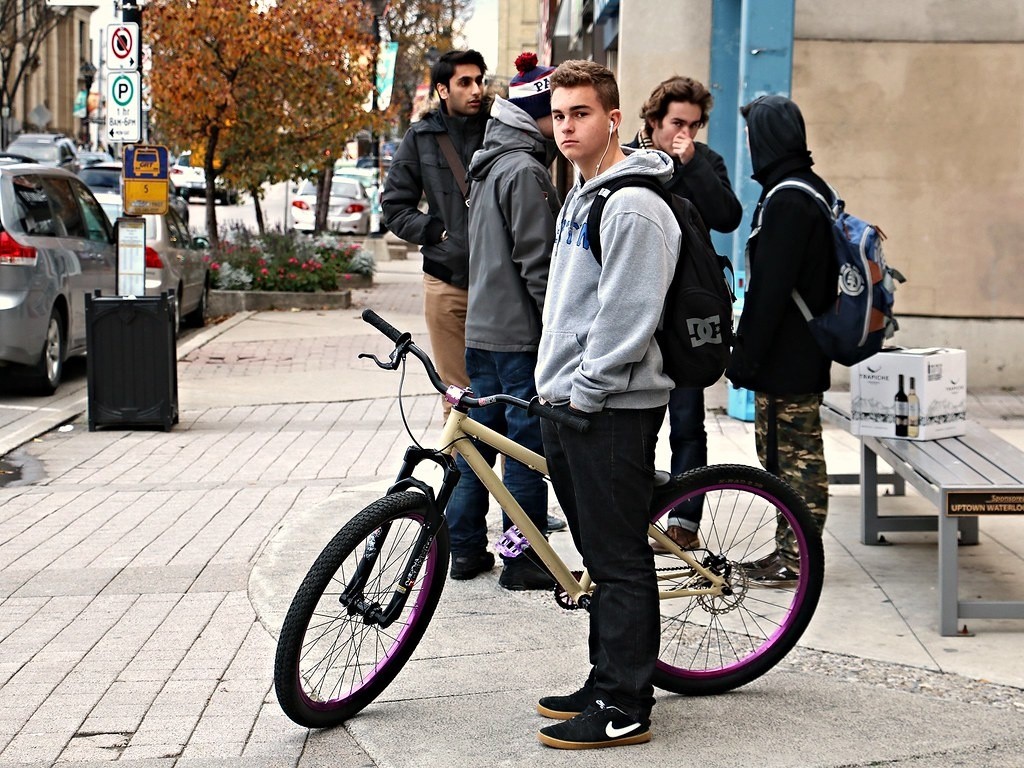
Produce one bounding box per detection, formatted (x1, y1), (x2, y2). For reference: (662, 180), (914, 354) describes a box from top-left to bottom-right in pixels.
(508, 52), (558, 119)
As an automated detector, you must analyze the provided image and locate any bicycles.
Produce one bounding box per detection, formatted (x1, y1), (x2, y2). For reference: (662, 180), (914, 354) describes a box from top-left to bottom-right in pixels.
(273, 310), (825, 729)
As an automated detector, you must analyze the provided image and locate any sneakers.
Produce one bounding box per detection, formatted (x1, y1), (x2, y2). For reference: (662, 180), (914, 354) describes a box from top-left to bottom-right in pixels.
(537, 683), (596, 720)
(499, 561), (556, 591)
(450, 551), (495, 580)
(730, 552), (800, 589)
(650, 525), (699, 554)
(537, 696), (651, 749)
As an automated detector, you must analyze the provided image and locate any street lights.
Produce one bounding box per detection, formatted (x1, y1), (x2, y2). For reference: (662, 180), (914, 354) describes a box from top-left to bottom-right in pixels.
(80, 62), (97, 152)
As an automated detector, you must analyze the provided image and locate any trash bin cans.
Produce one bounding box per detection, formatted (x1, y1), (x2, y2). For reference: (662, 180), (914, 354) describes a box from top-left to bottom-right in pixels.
(357, 134), (370, 158)
(82, 290), (180, 432)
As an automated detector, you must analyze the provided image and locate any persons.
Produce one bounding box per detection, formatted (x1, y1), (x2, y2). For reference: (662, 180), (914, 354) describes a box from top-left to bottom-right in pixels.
(723, 94), (842, 590)
(620, 75), (742, 554)
(533, 61), (684, 750)
(76, 140), (115, 159)
(448, 52), (567, 589)
(380, 50), (567, 532)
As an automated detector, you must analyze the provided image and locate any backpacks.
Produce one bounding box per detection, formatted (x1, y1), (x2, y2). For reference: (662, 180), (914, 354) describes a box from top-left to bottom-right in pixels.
(586, 173), (738, 390)
(744, 177), (906, 367)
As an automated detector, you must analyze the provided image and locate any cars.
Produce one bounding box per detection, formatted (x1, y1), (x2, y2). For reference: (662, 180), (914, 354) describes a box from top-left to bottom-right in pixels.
(0, 133), (81, 174)
(80, 194), (212, 335)
(334, 167), (388, 234)
(169, 149), (240, 205)
(289, 176), (370, 237)
(77, 162), (189, 225)
(355, 158), (394, 172)
(77, 152), (113, 165)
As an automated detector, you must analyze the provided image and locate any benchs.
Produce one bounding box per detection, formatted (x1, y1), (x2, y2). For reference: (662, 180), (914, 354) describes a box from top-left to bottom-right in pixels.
(819, 390), (1024, 637)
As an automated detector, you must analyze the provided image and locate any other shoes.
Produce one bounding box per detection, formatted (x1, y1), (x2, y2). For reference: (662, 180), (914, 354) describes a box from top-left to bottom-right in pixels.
(546, 515), (566, 532)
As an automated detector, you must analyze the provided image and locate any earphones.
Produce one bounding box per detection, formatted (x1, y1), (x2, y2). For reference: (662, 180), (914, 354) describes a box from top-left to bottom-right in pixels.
(609, 120), (614, 134)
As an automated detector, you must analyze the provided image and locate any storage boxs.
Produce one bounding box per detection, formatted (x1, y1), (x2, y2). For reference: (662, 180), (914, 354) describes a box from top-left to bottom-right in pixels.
(850, 345), (969, 441)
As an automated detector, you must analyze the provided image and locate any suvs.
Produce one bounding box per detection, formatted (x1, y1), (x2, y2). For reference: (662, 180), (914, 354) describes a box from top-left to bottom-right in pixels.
(0, 152), (118, 397)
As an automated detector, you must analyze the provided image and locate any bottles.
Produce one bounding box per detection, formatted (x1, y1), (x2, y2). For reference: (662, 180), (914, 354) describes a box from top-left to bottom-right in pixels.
(895, 375), (908, 437)
(908, 377), (919, 437)
